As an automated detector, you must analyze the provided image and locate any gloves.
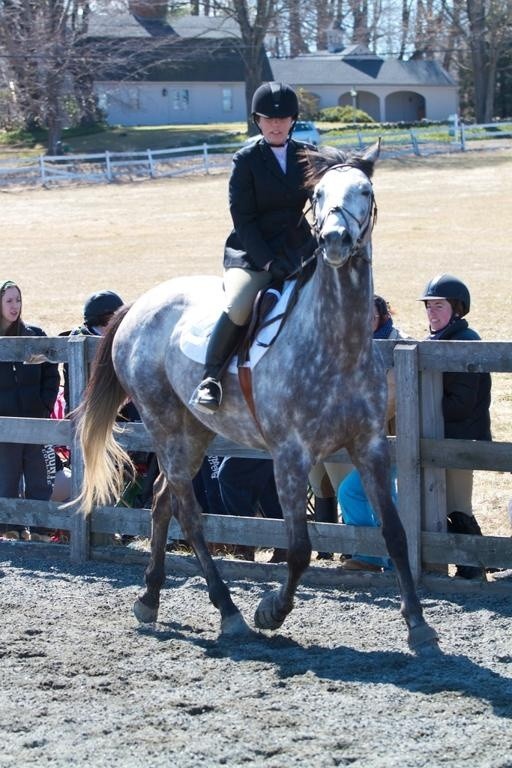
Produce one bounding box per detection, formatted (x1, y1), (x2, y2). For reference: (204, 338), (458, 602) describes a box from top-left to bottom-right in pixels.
(270, 260), (290, 280)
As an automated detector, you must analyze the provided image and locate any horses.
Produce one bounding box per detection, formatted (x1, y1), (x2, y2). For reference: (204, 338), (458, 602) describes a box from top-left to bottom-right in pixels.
(58, 135), (445, 652)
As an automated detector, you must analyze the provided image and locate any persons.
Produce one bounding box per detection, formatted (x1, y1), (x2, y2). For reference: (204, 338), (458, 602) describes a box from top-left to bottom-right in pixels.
(419, 275), (491, 580)
(188, 82), (321, 414)
(338, 463), (399, 571)
(307, 462), (355, 561)
(114, 401), (175, 547)
(372, 293), (418, 435)
(0, 280), (60, 544)
(59, 291), (142, 422)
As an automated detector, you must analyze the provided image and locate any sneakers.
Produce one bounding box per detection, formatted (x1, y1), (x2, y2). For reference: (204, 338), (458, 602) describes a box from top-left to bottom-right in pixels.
(194, 380), (220, 415)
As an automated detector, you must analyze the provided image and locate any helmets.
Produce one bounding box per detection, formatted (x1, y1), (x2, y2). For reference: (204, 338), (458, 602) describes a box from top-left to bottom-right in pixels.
(83, 290), (124, 316)
(250, 81), (299, 122)
(417, 272), (470, 316)
